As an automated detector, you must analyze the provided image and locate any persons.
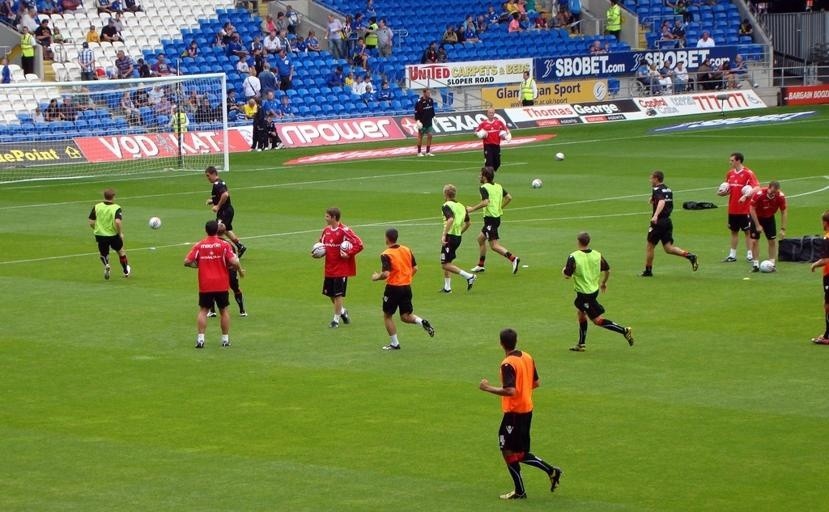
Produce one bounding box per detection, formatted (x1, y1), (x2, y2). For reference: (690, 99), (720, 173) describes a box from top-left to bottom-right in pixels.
(183, 221), (239, 347)
(479, 329), (562, 499)
(562, 232), (633, 351)
(465, 166), (520, 274)
(749, 181), (787, 271)
(642, 170), (698, 276)
(811, 210), (829, 344)
(89, 188), (131, 280)
(312, 209), (363, 328)
(717, 153), (759, 262)
(372, 229), (434, 351)
(209, 224), (248, 318)
(205, 166), (246, 257)
(438, 184), (476, 294)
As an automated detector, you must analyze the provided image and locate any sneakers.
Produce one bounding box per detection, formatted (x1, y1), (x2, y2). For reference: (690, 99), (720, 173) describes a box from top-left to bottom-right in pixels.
(642, 271), (652, 276)
(207, 310), (216, 318)
(240, 310), (247, 317)
(248, 143), (286, 152)
(624, 327), (633, 346)
(550, 468), (562, 492)
(195, 340), (204, 348)
(223, 340), (231, 346)
(416, 152), (435, 156)
(382, 343), (400, 350)
(422, 319), (434, 337)
(330, 309), (350, 327)
(437, 264), (485, 293)
(690, 255), (698, 271)
(234, 242), (246, 258)
(104, 263), (131, 279)
(512, 257), (520, 274)
(500, 490), (527, 499)
(721, 255), (776, 272)
(569, 344), (585, 351)
(811, 336), (829, 344)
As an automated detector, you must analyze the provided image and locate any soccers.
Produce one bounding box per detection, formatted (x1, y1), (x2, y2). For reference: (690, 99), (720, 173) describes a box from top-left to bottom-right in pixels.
(149, 216), (162, 229)
(340, 241), (354, 253)
(312, 243), (326, 258)
(479, 130), (488, 139)
(760, 260), (774, 272)
(556, 152), (565, 160)
(532, 178), (542, 189)
(499, 130), (507, 139)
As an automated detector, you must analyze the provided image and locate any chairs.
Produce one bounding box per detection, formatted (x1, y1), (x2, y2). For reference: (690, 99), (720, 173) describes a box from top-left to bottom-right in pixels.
(1, 1), (774, 148)
